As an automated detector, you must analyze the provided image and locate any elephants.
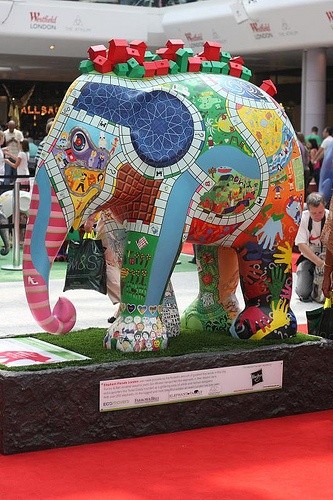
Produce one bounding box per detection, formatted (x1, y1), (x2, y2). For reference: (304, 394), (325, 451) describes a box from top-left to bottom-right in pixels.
(0, 189), (33, 256)
(23, 39), (304, 352)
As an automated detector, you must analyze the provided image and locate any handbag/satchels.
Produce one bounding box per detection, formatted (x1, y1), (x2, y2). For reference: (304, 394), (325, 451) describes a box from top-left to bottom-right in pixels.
(62, 229), (107, 295)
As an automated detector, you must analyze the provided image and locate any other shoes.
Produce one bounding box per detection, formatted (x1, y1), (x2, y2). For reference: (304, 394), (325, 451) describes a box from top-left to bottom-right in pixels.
(175, 260), (181, 264)
(188, 261), (196, 264)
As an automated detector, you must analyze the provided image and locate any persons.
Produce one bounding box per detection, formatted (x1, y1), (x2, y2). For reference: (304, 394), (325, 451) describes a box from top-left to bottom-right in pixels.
(294, 192), (329, 305)
(0, 120), (30, 195)
(35, 117), (54, 173)
(296, 126), (333, 200)
